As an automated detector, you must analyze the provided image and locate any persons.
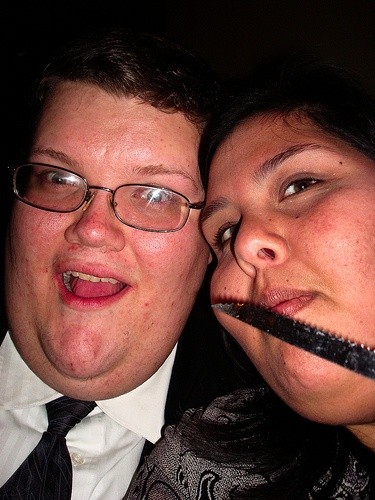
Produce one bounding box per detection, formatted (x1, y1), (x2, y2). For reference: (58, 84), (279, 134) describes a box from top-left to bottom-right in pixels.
(0, 23), (265, 500)
(123, 62), (375, 499)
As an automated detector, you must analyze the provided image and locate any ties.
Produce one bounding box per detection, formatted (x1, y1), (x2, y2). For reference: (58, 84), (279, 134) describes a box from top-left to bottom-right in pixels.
(0, 395), (97, 500)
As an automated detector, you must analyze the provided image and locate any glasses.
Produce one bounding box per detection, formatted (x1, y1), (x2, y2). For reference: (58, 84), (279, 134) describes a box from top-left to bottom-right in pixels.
(5, 162), (206, 234)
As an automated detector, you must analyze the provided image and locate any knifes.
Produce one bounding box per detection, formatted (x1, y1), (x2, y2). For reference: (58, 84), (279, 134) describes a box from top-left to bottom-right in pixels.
(211, 301), (375, 382)
